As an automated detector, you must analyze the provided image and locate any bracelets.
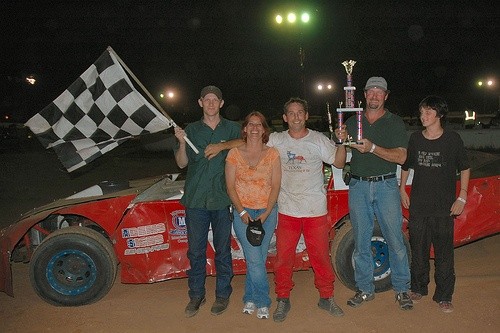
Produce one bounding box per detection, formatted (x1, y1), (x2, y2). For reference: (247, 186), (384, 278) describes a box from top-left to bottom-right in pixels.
(238, 210), (247, 217)
(461, 188), (468, 193)
(457, 196), (466, 204)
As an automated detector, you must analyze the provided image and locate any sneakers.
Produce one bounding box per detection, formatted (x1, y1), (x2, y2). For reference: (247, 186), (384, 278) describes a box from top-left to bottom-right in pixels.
(317, 296), (345, 317)
(273, 300), (291, 321)
(256, 306), (271, 319)
(409, 291), (424, 300)
(242, 301), (257, 315)
(347, 289), (376, 308)
(395, 292), (414, 310)
(439, 296), (456, 312)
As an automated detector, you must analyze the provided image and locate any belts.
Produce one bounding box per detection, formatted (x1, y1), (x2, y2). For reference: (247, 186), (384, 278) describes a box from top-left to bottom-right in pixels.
(351, 173), (397, 182)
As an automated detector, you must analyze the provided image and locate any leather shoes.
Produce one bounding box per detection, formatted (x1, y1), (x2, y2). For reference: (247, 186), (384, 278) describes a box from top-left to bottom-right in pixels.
(184, 296), (206, 318)
(210, 296), (231, 315)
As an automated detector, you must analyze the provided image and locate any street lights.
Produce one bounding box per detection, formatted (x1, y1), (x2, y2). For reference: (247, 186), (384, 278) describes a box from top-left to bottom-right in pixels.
(272, 9), (313, 101)
(478, 80), (492, 115)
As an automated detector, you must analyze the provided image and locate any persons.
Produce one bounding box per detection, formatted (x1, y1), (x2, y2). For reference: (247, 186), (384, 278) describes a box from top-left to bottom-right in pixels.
(462, 105), (476, 129)
(172, 86), (247, 318)
(204, 97), (349, 323)
(225, 112), (282, 319)
(399, 96), (471, 314)
(322, 77), (413, 311)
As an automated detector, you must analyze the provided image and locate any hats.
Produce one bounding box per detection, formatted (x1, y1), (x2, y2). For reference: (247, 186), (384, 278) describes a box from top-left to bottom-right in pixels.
(201, 85), (223, 99)
(246, 219), (265, 246)
(365, 77), (388, 92)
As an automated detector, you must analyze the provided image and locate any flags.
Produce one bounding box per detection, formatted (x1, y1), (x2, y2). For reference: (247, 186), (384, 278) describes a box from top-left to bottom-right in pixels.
(23, 48), (172, 172)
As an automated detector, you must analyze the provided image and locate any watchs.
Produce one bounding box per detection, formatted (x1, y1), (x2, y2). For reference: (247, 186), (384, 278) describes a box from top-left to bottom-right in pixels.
(369, 144), (376, 153)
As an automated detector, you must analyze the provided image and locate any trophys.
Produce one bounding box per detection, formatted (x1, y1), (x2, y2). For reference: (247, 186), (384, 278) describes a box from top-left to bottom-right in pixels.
(334, 60), (364, 146)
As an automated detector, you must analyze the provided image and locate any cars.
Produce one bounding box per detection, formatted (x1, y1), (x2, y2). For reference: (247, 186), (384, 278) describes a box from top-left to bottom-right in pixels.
(0, 131), (500, 307)
